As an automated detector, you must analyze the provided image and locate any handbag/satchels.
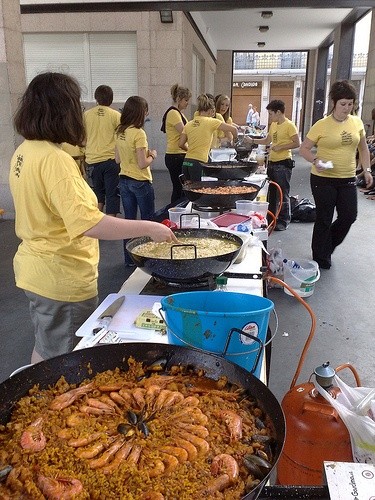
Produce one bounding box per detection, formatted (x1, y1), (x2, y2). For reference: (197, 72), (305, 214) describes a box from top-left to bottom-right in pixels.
(314, 374), (375, 463)
(292, 197), (317, 222)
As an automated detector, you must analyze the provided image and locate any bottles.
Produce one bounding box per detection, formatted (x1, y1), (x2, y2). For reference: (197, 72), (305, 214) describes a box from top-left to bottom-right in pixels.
(213, 276), (228, 292)
(283, 258), (303, 269)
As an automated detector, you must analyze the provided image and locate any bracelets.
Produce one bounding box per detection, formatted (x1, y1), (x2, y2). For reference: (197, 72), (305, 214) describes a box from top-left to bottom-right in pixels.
(313, 157), (317, 165)
(252, 138), (254, 143)
(147, 154), (154, 159)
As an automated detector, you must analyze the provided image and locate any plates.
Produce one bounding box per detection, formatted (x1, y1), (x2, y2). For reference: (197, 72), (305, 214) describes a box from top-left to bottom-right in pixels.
(211, 212), (252, 227)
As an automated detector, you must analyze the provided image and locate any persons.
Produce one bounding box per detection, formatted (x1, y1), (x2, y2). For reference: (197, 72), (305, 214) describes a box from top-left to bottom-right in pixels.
(214, 94), (239, 149)
(9, 72), (180, 365)
(179, 95), (238, 181)
(194, 93), (226, 148)
(115, 96), (157, 266)
(355, 108), (375, 200)
(299, 80), (373, 269)
(160, 83), (192, 204)
(81, 84), (122, 218)
(245, 103), (260, 127)
(57, 142), (84, 178)
(243, 99), (300, 231)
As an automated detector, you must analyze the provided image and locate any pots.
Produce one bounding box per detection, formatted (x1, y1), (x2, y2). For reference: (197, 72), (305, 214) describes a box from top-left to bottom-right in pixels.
(235, 147), (252, 159)
(125, 214), (244, 282)
(179, 173), (268, 207)
(0, 342), (287, 499)
(200, 154), (259, 180)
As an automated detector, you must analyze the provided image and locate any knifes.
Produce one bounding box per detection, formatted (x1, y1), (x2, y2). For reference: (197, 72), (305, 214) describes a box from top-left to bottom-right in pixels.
(93, 296), (125, 335)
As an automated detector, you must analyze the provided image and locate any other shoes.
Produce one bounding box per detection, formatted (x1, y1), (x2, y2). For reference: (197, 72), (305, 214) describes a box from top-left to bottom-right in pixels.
(275, 224), (289, 231)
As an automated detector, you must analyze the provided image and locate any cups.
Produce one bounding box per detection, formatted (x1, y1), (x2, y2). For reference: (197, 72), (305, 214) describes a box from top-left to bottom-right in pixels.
(235, 199), (253, 215)
(253, 201), (269, 219)
(168, 207), (187, 229)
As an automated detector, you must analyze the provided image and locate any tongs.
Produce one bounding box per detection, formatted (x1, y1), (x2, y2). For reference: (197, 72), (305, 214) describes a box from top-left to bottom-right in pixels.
(74, 292), (170, 344)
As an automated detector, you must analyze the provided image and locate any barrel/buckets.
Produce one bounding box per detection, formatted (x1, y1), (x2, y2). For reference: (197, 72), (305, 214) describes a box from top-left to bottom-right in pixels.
(284, 258), (320, 297)
(208, 149), (238, 162)
(158, 290), (279, 380)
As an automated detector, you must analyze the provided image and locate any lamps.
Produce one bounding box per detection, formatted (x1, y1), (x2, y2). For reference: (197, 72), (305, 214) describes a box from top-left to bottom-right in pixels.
(159, 8), (173, 23)
